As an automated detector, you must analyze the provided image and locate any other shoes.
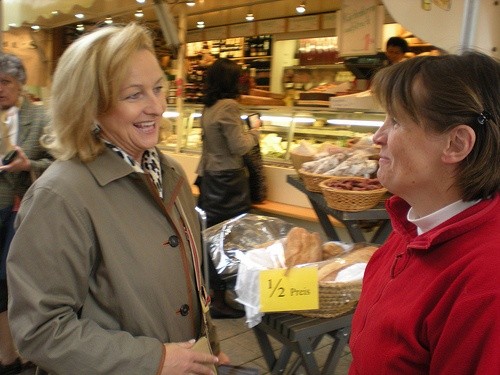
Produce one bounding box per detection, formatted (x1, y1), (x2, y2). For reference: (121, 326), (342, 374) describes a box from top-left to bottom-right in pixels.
(0, 356), (34, 375)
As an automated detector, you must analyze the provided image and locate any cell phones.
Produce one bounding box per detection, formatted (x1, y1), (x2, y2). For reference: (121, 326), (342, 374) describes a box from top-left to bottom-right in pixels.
(3, 150), (16, 164)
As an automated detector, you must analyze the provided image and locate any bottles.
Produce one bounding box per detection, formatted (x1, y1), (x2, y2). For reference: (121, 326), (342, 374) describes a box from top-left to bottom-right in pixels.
(186, 36), (273, 101)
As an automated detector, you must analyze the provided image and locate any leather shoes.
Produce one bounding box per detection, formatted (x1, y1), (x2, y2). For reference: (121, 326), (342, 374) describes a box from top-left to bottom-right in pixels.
(212, 306), (245, 318)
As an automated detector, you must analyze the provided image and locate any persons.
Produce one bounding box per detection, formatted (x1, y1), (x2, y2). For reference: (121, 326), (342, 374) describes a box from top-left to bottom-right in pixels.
(7, 20), (229, 375)
(0, 51), (56, 375)
(348, 49), (500, 375)
(386, 37), (408, 63)
(195, 58), (261, 228)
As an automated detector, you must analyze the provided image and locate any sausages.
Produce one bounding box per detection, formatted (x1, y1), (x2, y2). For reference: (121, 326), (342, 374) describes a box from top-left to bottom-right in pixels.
(301, 137), (383, 191)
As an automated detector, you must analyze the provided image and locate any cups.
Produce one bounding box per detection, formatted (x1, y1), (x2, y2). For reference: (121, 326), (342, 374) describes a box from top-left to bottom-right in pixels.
(248, 114), (261, 129)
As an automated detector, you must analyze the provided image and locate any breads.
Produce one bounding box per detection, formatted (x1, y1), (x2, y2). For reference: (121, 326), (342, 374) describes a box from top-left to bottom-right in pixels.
(283, 227), (379, 267)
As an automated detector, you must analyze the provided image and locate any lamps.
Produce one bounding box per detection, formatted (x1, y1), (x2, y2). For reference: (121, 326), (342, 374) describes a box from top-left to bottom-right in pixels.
(31, 0), (308, 32)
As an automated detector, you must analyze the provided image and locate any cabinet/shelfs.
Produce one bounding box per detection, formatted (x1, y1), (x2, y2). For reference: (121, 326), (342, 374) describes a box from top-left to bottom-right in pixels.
(184, 33), (272, 104)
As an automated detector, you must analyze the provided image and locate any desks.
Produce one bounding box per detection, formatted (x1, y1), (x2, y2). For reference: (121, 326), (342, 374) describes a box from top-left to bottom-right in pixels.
(221, 172), (393, 375)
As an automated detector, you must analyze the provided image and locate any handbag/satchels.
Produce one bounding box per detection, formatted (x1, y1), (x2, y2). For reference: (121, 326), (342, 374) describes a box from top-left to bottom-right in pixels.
(196, 168), (248, 215)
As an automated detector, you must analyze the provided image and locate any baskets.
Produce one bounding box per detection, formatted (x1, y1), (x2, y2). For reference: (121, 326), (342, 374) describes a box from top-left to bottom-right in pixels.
(298, 169), (333, 192)
(277, 283), (363, 320)
(319, 177), (388, 210)
(346, 139), (382, 160)
(289, 153), (327, 178)
